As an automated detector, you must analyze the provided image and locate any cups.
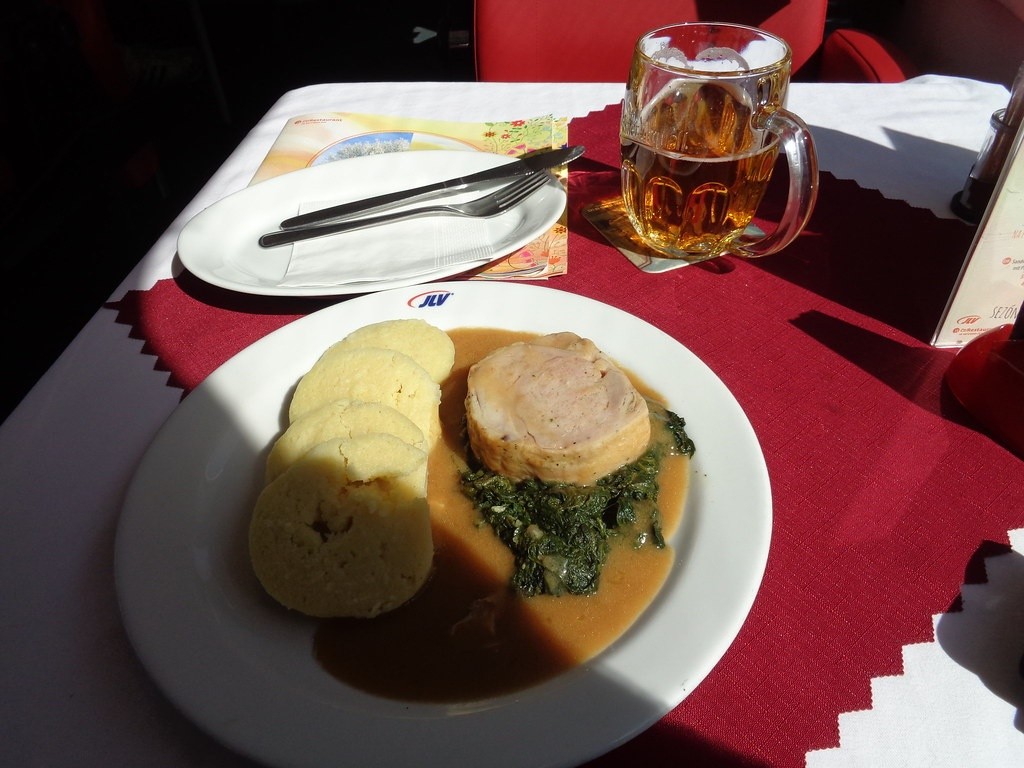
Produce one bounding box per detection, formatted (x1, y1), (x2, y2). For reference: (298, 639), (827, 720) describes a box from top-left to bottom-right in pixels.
(620, 22), (819, 258)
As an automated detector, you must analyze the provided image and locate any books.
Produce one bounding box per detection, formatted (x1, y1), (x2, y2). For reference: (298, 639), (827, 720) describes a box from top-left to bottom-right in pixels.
(247, 114), (569, 280)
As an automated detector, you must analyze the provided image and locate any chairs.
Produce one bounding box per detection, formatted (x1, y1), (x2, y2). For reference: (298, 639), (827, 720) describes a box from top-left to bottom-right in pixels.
(471, 0), (911, 83)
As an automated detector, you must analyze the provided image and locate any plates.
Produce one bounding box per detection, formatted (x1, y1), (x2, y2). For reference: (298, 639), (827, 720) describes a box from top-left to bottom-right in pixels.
(176, 150), (567, 296)
(113, 282), (773, 768)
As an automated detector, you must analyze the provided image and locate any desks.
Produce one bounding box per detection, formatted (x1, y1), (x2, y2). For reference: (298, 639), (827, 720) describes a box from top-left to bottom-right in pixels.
(0, 73), (1024, 768)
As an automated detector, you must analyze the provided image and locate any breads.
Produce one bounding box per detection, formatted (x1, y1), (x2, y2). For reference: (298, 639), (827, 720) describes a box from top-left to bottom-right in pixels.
(247, 319), (456, 618)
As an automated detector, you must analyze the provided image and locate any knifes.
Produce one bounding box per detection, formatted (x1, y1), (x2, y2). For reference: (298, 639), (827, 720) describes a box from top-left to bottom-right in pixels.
(279, 146), (588, 232)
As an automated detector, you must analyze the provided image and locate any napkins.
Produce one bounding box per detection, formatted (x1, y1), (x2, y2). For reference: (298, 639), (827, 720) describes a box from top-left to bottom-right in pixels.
(275, 182), (494, 287)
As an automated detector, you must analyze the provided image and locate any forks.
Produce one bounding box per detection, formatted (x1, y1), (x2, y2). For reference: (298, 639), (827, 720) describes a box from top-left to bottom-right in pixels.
(258, 167), (553, 248)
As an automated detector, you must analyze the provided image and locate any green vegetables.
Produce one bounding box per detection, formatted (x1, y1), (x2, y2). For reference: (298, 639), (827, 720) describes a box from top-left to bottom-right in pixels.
(459, 399), (695, 599)
